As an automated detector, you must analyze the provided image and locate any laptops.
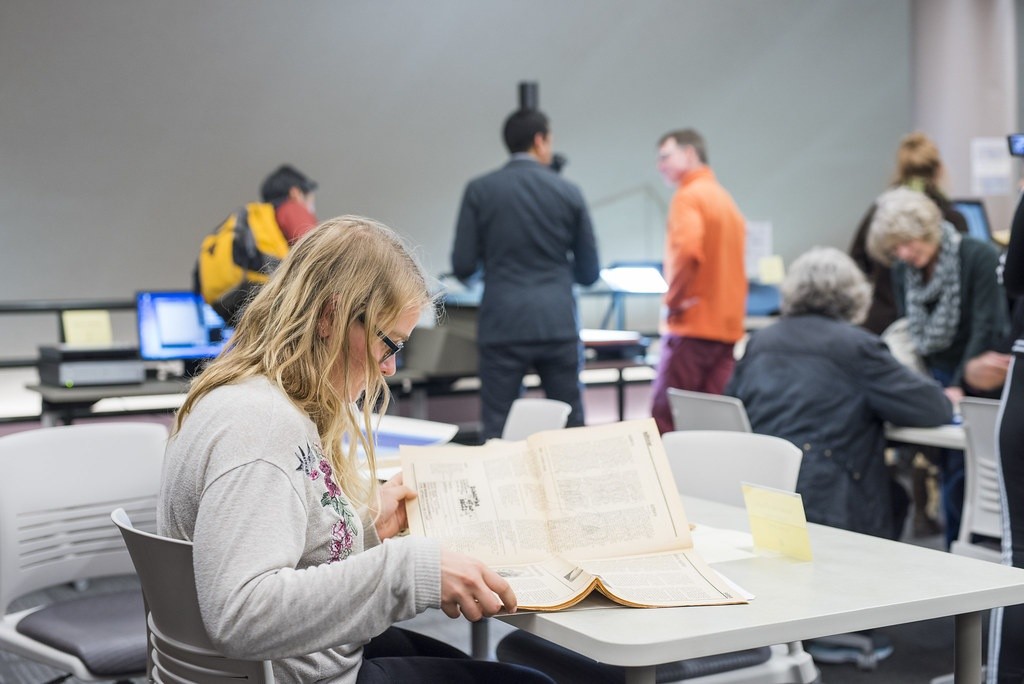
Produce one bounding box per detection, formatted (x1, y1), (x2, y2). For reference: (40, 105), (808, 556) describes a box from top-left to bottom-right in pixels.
(951, 200), (993, 245)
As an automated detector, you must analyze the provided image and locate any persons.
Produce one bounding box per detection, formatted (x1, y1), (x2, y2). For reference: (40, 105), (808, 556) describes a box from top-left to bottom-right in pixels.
(156, 215), (558, 684)
(854, 135), (970, 335)
(451, 107), (600, 439)
(723, 244), (963, 664)
(986, 190), (1024, 684)
(651, 129), (746, 434)
(260, 164), (323, 246)
(867, 186), (1012, 551)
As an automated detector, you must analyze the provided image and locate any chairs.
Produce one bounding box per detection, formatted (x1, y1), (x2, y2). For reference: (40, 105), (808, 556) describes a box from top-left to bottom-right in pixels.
(502, 386), (878, 684)
(0, 419), (276, 684)
(932, 397), (1003, 683)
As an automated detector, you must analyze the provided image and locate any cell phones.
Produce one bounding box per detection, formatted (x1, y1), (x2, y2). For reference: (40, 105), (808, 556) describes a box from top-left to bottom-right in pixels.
(1007, 134), (1024, 156)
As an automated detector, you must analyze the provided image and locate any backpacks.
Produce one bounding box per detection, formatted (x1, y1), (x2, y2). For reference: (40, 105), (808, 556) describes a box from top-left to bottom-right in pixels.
(198, 202), (291, 326)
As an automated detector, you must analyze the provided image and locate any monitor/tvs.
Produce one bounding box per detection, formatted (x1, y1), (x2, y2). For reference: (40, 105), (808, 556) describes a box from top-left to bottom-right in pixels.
(135, 290), (237, 377)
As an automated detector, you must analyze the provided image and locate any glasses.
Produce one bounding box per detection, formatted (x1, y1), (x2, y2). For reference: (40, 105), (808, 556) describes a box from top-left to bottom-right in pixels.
(355, 306), (402, 363)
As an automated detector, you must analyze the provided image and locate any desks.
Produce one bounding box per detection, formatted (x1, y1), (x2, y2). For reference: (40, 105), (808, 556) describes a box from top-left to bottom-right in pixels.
(882, 420), (967, 450)
(446, 440), (1024, 684)
(26, 359), (656, 430)
